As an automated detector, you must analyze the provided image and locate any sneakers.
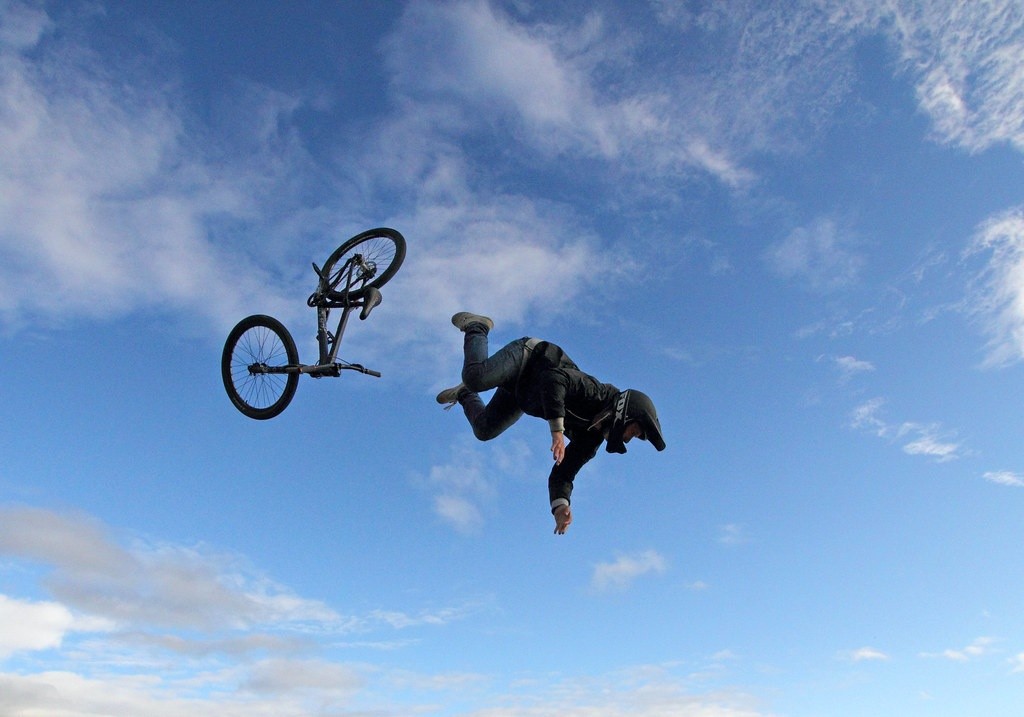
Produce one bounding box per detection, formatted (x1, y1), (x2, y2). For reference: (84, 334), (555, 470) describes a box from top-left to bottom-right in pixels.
(451, 312), (493, 332)
(436, 383), (468, 411)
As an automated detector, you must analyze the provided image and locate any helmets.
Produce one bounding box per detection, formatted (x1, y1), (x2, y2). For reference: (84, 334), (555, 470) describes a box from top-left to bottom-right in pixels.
(606, 389), (666, 454)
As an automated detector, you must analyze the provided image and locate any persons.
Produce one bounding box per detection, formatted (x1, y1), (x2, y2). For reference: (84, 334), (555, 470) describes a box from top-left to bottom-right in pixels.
(437, 312), (666, 534)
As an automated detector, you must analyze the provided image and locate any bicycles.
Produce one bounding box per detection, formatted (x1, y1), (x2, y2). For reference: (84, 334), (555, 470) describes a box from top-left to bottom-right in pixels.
(222, 227), (406, 419)
